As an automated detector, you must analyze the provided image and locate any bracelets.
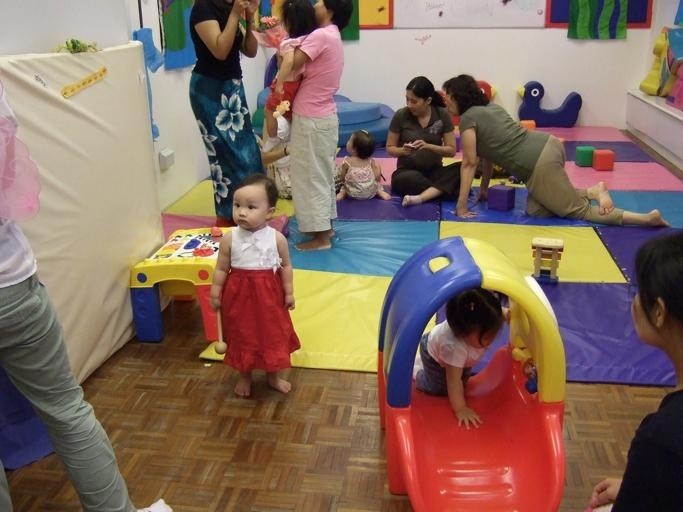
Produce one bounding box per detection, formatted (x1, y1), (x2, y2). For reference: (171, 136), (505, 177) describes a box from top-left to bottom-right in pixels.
(284, 146), (288, 155)
(246, 20), (255, 24)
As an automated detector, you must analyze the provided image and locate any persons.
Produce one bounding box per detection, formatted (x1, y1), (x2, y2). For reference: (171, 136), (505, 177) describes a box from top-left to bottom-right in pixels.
(336, 130), (392, 202)
(260, 113), (342, 199)
(386, 75), (462, 209)
(412, 287), (511, 429)
(0, 78), (139, 512)
(442, 74), (670, 228)
(208, 173), (301, 398)
(261, 1), (318, 153)
(586, 228), (683, 512)
(188, 1), (265, 228)
(272, 1), (355, 251)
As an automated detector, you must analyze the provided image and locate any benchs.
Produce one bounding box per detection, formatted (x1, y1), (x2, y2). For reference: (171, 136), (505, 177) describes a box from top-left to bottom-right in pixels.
(627, 90), (683, 170)
